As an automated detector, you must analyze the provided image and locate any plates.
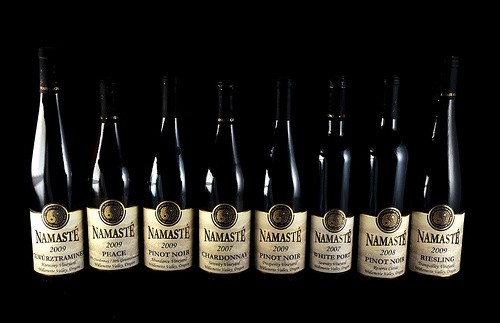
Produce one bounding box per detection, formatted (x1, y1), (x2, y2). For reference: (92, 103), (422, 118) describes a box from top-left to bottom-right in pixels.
(358, 76), (411, 282)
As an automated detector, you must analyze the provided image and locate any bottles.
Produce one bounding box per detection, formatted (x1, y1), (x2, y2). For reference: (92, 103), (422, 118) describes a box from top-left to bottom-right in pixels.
(197, 80), (253, 282)
(310, 77), (360, 279)
(86, 78), (141, 276)
(408, 56), (466, 281)
(141, 75), (197, 277)
(253, 80), (310, 279)
(28, 47), (87, 281)
